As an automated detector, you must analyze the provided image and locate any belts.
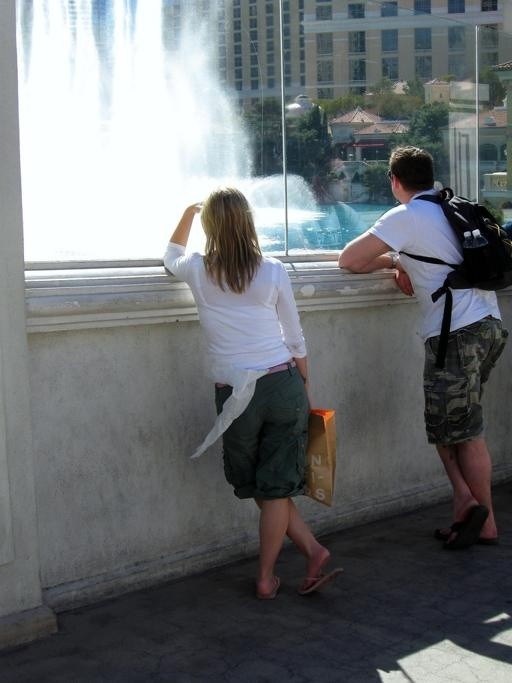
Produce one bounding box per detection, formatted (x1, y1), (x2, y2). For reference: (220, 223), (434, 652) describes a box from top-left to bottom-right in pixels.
(216, 361), (297, 388)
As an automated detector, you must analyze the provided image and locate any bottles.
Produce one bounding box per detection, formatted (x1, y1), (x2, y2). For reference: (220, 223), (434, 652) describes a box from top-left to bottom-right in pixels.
(472, 229), (488, 247)
(462, 230), (474, 250)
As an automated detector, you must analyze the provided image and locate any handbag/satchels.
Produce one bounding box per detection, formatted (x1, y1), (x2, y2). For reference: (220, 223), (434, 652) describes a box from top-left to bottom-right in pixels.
(304, 408), (337, 507)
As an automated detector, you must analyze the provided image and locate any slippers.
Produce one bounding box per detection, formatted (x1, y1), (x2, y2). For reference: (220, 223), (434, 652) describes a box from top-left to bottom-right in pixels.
(434, 504), (499, 550)
(297, 568), (344, 595)
(256, 577), (281, 600)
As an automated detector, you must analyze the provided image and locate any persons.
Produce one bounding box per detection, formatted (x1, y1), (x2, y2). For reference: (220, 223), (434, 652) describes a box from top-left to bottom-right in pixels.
(165, 186), (344, 602)
(340, 146), (506, 546)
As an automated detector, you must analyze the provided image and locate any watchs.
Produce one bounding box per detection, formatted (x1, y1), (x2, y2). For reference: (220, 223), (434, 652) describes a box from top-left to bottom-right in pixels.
(389, 253), (398, 269)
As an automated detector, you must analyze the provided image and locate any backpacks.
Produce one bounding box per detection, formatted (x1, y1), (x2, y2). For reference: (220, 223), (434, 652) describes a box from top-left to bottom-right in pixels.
(399, 194), (512, 290)
